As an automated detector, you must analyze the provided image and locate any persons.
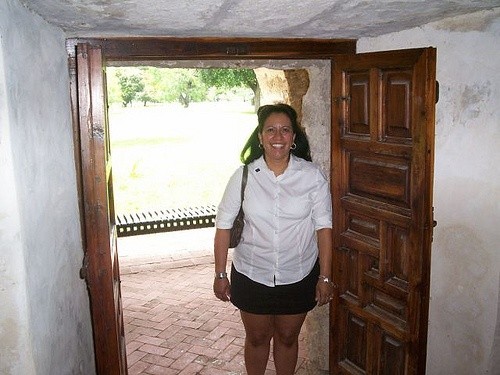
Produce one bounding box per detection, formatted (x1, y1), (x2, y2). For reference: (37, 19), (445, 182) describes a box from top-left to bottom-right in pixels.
(213, 104), (334, 375)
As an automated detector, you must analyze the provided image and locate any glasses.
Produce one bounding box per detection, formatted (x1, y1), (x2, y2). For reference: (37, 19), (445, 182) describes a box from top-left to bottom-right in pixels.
(264, 126), (292, 136)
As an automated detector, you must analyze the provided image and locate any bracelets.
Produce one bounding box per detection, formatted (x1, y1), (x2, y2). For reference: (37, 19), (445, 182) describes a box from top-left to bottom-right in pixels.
(318, 274), (331, 285)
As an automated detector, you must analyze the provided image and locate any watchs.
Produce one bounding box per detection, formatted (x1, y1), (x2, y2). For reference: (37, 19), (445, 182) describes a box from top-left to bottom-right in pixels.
(214, 271), (227, 280)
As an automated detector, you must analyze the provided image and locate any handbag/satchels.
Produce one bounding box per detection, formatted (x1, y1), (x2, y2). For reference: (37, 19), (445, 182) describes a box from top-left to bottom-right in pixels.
(229, 165), (248, 248)
